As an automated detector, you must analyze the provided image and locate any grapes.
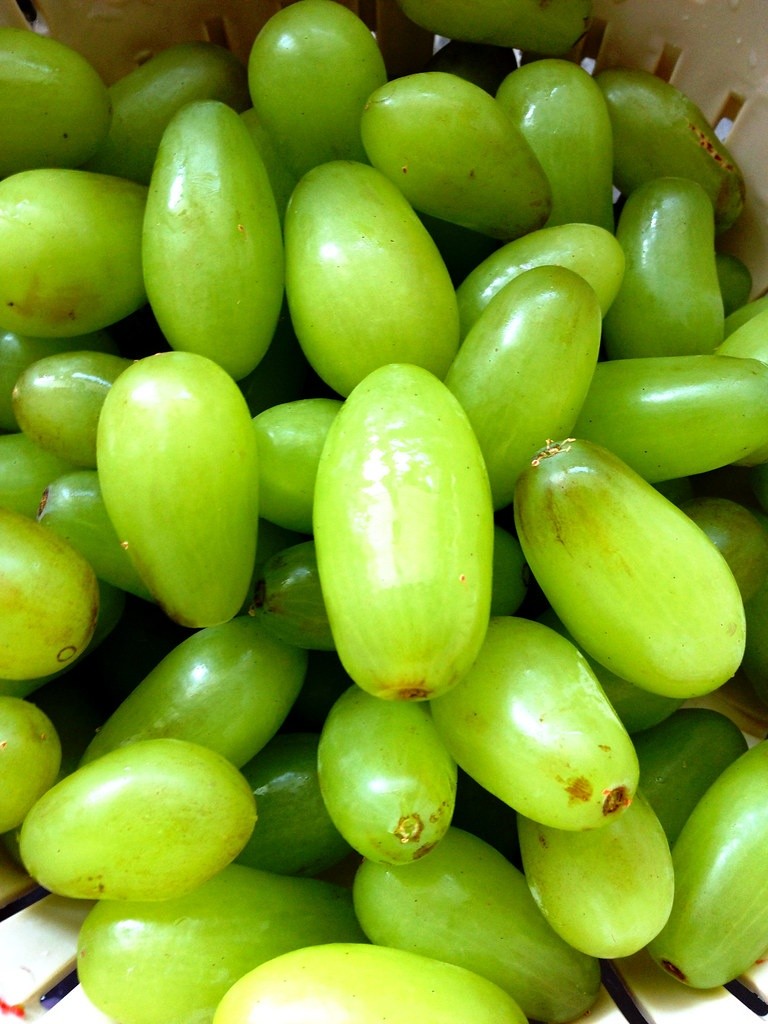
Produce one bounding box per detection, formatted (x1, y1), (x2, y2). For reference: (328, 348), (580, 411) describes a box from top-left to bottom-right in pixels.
(0, 0), (768, 1024)
